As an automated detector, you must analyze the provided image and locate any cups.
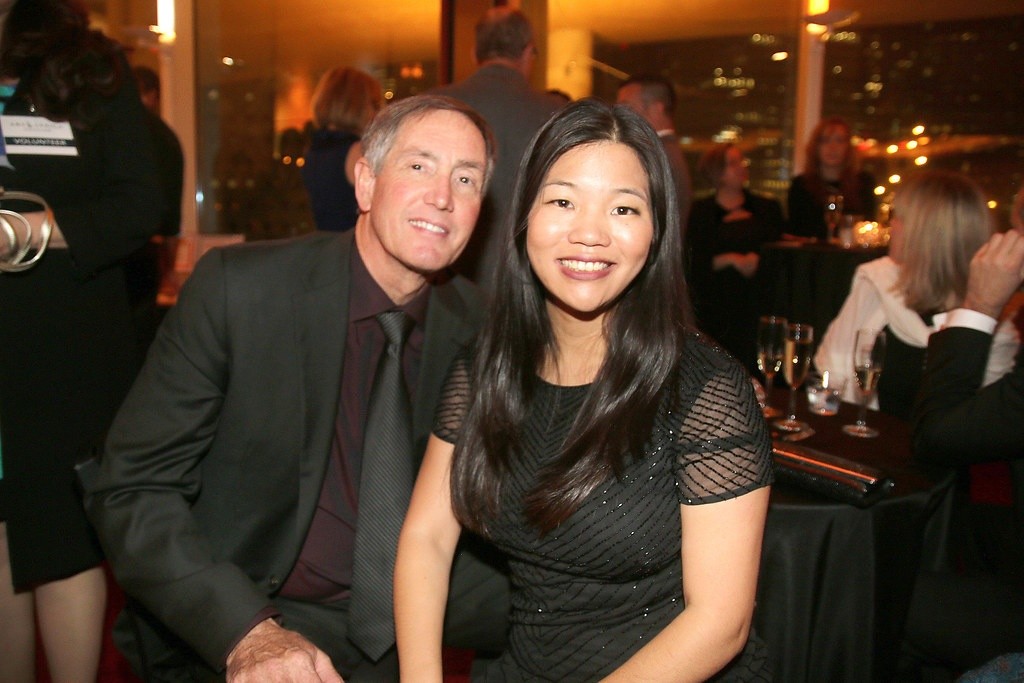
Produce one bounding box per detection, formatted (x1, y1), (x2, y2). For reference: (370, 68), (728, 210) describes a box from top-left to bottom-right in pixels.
(806, 371), (848, 415)
(840, 215), (863, 246)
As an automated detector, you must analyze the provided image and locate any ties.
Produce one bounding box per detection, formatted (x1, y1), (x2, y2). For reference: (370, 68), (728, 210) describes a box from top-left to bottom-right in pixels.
(345, 312), (415, 661)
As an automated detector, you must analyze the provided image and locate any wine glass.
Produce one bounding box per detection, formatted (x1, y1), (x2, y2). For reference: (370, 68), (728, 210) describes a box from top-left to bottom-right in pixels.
(775, 323), (813, 431)
(826, 195), (843, 245)
(844, 328), (886, 437)
(753, 314), (789, 417)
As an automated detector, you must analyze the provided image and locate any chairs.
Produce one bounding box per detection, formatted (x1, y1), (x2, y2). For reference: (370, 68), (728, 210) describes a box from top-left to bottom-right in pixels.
(757, 450), (892, 683)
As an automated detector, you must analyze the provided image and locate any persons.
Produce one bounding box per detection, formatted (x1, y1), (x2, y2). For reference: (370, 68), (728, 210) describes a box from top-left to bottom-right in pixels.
(96, 94), (510, 683)
(1, 0), (157, 683)
(678, 143), (783, 358)
(618, 69), (692, 244)
(811, 168), (1022, 581)
(782, 125), (882, 332)
(131, 65), (183, 374)
(395, 97), (777, 683)
(306, 63), (386, 231)
(419, 6), (569, 286)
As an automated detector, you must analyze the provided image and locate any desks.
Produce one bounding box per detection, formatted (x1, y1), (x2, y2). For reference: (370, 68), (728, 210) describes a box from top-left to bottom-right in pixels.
(754, 383), (918, 500)
(766, 237), (890, 341)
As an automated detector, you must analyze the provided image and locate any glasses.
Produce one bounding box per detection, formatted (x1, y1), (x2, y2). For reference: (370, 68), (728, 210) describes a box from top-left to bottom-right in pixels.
(528, 45), (542, 62)
